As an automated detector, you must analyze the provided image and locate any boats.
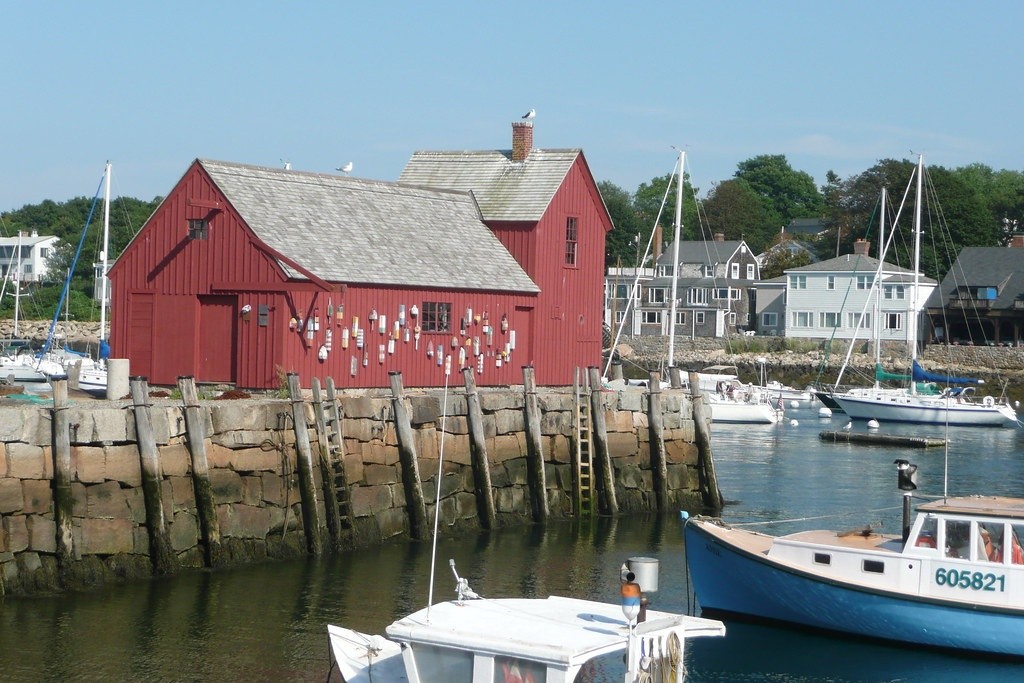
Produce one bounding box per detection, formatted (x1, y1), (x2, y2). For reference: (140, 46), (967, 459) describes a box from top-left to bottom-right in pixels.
(752, 360), (818, 402)
(326, 376), (726, 683)
(680, 342), (1024, 664)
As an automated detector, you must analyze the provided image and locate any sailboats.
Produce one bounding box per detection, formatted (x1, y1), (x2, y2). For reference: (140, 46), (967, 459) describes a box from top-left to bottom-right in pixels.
(0, 157), (114, 399)
(813, 149), (1017, 427)
(600, 138), (785, 425)
